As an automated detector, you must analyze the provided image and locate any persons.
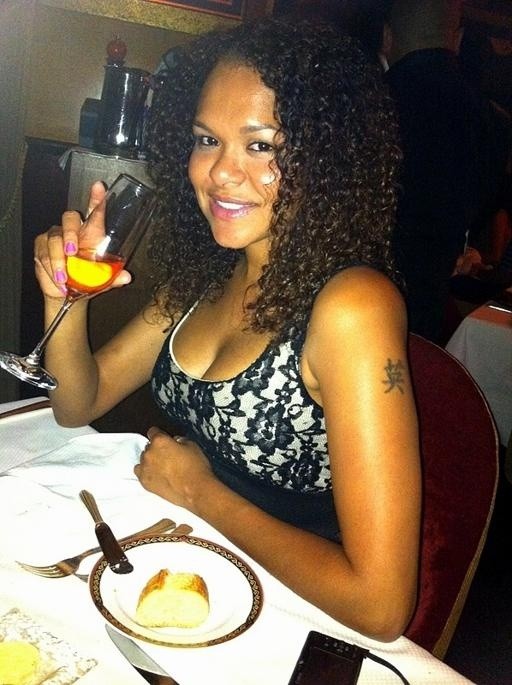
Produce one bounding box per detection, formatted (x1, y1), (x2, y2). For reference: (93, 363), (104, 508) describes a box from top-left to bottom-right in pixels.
(437, 246), (492, 306)
(31, 10), (424, 643)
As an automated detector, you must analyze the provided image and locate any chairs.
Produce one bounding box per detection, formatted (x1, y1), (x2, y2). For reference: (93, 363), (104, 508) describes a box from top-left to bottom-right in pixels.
(405, 330), (499, 662)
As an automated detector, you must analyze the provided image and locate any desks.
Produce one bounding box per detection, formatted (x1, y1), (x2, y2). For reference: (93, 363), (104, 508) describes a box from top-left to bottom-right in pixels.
(22, 136), (158, 432)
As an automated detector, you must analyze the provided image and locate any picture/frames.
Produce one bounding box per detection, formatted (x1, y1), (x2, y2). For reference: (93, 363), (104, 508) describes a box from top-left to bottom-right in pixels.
(40, 0), (276, 37)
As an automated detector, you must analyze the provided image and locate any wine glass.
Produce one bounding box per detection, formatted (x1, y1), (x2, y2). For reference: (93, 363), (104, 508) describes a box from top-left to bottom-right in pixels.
(0, 172), (159, 390)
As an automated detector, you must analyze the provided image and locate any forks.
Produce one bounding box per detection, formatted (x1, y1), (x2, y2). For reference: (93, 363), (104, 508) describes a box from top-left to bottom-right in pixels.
(15, 519), (176, 577)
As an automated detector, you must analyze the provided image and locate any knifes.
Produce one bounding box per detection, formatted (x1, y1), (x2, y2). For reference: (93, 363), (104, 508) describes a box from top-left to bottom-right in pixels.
(105, 623), (178, 685)
(79, 489), (134, 574)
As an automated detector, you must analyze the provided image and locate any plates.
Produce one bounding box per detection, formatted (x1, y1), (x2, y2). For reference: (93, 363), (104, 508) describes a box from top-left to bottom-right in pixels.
(88, 532), (264, 650)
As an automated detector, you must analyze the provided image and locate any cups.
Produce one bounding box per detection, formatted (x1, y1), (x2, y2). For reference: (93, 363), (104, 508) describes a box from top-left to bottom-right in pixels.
(93, 65), (152, 159)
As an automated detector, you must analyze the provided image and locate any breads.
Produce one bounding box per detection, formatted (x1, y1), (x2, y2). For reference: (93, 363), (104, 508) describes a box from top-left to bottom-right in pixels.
(137, 568), (211, 627)
(0, 638), (39, 684)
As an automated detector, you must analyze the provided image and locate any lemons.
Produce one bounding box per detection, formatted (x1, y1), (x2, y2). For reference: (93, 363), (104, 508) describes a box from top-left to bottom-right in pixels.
(66, 256), (111, 287)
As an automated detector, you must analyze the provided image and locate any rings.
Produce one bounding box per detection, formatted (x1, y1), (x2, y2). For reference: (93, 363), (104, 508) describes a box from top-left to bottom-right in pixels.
(175, 436), (188, 443)
